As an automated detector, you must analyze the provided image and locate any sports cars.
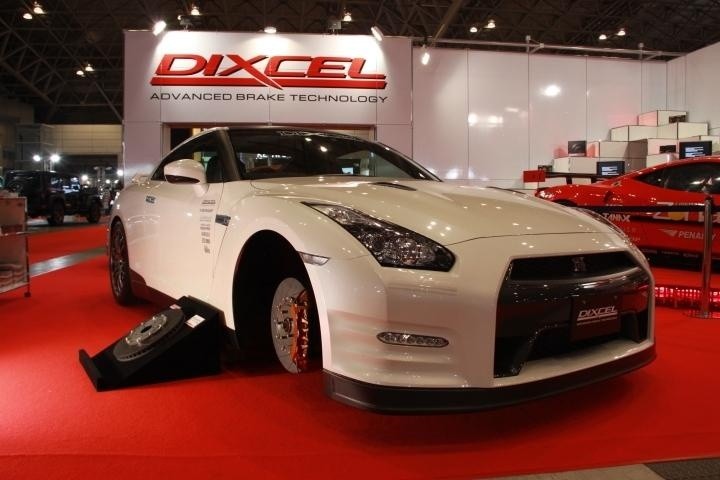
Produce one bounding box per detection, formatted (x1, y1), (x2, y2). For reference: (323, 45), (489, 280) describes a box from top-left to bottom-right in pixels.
(106, 125), (659, 417)
(534, 155), (720, 263)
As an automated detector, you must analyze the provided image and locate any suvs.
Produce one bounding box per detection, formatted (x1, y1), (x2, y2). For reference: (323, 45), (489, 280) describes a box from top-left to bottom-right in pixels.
(2, 167), (104, 228)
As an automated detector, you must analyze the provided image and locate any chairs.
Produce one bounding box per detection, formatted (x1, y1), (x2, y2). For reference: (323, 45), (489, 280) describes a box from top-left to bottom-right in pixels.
(206, 140), (343, 183)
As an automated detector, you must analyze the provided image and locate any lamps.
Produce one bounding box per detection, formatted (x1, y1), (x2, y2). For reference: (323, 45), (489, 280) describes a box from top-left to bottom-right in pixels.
(150, 20), (166, 36)
(22, 1), (45, 21)
(264, 26), (277, 34)
(616, 23), (626, 36)
(468, 24), (478, 33)
(343, 7), (351, 22)
(598, 30), (607, 40)
(190, 1), (201, 17)
(72, 59), (95, 76)
(371, 25), (384, 41)
(421, 23), (446, 66)
(487, 17), (496, 29)
